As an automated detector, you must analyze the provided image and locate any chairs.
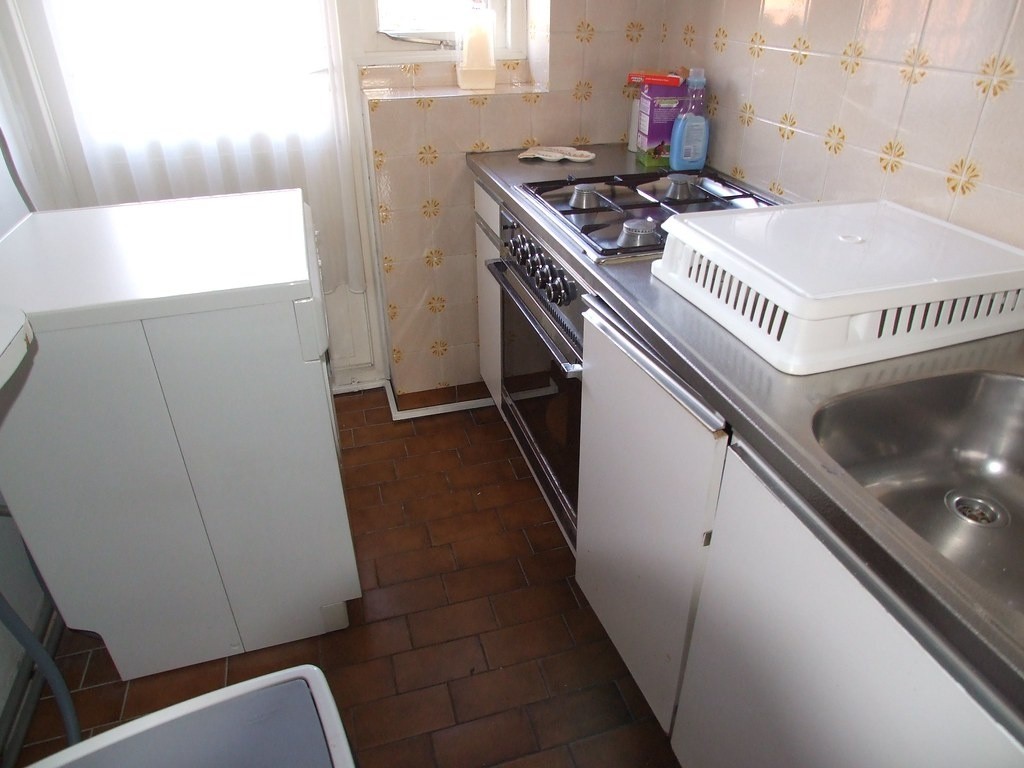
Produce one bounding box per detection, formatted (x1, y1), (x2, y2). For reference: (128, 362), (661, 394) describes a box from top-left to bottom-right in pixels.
(0, 591), (357, 768)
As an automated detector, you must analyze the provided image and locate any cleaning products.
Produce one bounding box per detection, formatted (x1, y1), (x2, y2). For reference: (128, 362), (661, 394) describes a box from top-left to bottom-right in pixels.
(669, 68), (710, 170)
(455, 0), (497, 90)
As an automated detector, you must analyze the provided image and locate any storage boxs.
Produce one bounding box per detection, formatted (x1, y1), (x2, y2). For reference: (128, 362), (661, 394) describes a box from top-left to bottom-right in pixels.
(651, 197), (1024, 375)
(627, 71), (707, 168)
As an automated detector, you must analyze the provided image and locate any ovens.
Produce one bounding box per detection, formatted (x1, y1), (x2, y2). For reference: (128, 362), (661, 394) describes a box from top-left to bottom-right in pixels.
(485, 202), (598, 553)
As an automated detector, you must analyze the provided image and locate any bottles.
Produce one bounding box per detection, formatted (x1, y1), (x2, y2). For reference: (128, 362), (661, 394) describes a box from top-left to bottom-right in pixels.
(669, 67), (710, 172)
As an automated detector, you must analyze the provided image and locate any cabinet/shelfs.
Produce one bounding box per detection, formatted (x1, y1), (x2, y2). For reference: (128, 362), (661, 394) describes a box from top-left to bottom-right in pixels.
(573, 294), (1024, 768)
(0, 187), (363, 686)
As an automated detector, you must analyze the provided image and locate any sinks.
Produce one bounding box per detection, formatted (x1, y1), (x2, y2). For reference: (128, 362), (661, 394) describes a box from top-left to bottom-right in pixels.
(810, 369), (1024, 617)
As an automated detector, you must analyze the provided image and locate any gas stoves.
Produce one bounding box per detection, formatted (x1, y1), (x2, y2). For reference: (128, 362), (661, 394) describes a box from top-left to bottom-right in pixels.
(512, 168), (785, 264)
